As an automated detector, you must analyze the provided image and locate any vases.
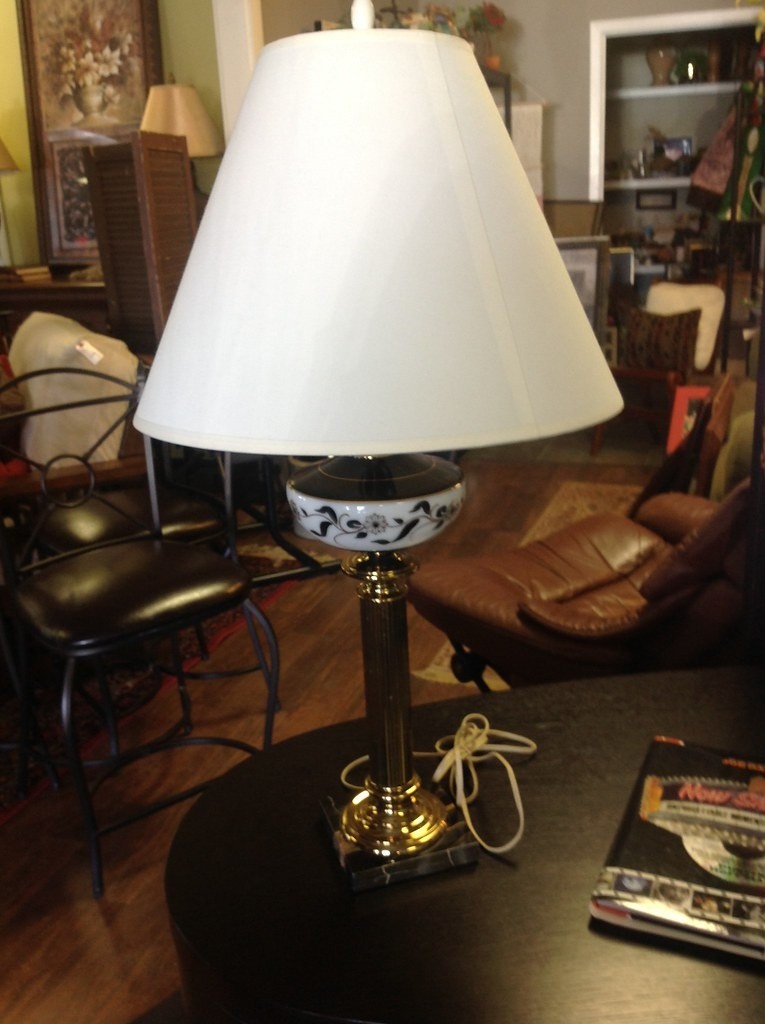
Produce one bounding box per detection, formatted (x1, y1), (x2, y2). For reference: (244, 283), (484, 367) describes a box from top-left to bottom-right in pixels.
(482, 55), (500, 70)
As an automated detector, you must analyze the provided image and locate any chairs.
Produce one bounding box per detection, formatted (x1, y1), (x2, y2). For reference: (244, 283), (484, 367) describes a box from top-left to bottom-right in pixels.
(591, 284), (727, 456)
(0, 367), (285, 897)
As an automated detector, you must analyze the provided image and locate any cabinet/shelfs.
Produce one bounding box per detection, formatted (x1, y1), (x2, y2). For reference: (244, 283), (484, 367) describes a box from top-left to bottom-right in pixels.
(588, 6), (765, 203)
(0, 262), (110, 347)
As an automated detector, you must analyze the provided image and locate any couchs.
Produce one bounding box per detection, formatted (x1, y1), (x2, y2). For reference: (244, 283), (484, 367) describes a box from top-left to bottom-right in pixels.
(405, 410), (765, 693)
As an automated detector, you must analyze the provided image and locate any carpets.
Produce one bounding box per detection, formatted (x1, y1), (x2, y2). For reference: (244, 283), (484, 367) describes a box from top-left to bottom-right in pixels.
(0, 544), (336, 823)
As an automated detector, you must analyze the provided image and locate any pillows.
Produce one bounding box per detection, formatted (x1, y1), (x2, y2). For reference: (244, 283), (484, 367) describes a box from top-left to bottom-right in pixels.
(615, 307), (699, 382)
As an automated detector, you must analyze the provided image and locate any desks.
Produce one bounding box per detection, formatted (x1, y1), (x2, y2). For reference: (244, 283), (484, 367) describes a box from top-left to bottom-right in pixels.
(166, 667), (765, 1024)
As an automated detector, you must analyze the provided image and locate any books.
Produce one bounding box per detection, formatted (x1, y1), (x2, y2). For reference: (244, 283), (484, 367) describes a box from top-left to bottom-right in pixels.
(664, 385), (712, 457)
(587, 732), (764, 963)
(0, 264), (53, 284)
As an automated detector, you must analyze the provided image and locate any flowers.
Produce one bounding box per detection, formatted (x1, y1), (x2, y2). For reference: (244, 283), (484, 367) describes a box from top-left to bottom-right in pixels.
(379, 0), (507, 55)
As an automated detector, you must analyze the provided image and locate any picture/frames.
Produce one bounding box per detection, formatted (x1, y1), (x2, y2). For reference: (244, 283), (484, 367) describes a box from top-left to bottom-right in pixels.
(16, 0), (166, 273)
(554, 230), (696, 366)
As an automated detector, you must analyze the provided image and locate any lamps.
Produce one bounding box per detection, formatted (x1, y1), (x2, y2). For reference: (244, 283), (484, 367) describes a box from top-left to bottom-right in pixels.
(132, 28), (627, 892)
(140, 72), (225, 193)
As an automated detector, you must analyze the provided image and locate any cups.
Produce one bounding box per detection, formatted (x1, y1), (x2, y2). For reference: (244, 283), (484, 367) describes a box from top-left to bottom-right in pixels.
(646, 49), (676, 84)
